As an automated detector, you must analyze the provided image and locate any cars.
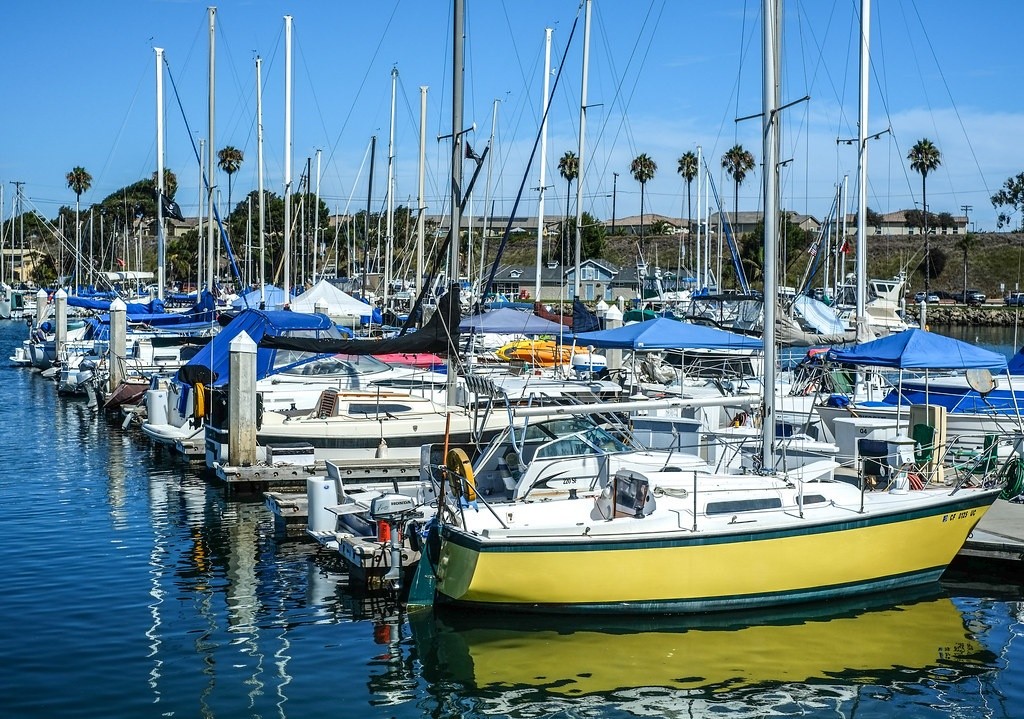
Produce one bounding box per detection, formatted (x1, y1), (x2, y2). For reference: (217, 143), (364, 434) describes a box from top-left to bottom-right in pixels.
(927, 293), (940, 303)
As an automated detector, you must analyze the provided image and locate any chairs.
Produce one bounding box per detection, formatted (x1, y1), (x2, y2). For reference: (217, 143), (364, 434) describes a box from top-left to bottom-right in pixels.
(910, 424), (936, 480)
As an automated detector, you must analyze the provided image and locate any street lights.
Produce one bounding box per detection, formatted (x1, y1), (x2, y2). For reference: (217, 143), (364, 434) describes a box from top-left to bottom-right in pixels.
(612, 171), (619, 236)
(915, 201), (929, 212)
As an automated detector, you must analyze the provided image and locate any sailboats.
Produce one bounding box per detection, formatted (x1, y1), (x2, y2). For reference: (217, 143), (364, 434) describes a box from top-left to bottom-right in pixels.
(0, 0), (1024, 607)
(405, 593), (996, 719)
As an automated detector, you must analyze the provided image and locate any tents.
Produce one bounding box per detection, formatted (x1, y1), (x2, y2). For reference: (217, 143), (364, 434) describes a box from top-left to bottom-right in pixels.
(232, 284), (295, 311)
(456, 307), (569, 379)
(825, 328), (1022, 436)
(290, 279), (373, 337)
(554, 318), (765, 398)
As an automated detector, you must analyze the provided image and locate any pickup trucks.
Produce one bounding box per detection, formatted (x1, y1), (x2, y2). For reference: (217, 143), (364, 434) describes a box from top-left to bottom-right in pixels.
(953, 288), (987, 304)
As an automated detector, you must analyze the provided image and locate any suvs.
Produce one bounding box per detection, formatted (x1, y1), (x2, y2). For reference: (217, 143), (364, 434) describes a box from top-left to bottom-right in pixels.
(1005, 292), (1024, 307)
(914, 291), (927, 303)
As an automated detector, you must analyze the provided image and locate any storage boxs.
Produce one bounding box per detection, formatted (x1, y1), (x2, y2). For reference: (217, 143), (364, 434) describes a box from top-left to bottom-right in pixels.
(265, 441), (315, 466)
